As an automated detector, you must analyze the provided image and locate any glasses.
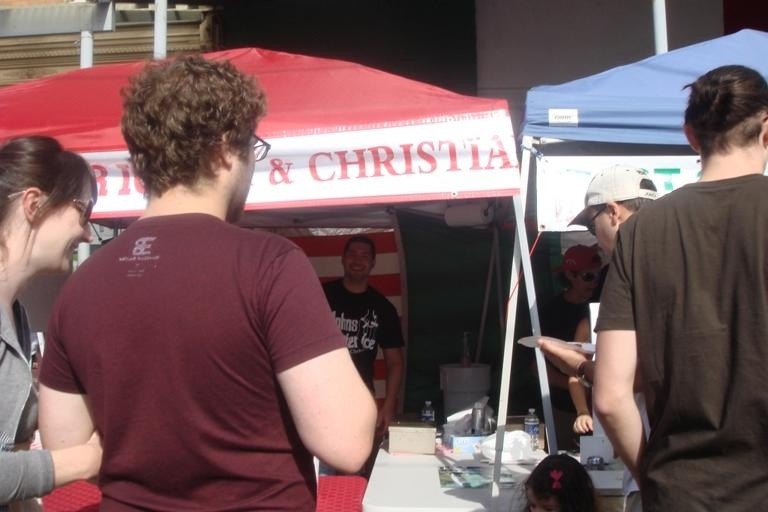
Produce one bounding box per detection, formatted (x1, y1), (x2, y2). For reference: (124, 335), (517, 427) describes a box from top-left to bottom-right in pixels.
(7, 190), (94, 226)
(580, 272), (594, 282)
(252, 134), (271, 161)
(585, 206), (607, 235)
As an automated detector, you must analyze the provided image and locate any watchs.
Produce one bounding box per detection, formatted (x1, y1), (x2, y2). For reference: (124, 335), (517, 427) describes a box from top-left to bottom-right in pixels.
(577, 360), (594, 388)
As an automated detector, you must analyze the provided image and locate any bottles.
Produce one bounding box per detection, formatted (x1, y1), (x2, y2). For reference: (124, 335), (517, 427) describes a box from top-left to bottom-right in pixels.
(421, 401), (435, 422)
(524, 409), (539, 452)
(587, 455), (605, 471)
(461, 336), (472, 367)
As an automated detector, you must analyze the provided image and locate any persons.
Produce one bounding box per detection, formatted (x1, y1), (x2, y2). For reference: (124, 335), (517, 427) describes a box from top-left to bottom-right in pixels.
(569, 263), (610, 456)
(37, 53), (378, 512)
(525, 453), (603, 511)
(528, 245), (602, 455)
(318, 236), (404, 477)
(537, 164), (660, 512)
(0, 133), (105, 512)
(592, 65), (768, 512)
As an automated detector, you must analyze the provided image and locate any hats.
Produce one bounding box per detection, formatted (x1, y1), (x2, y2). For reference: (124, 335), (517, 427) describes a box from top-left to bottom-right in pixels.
(567, 164), (658, 227)
(552, 244), (602, 272)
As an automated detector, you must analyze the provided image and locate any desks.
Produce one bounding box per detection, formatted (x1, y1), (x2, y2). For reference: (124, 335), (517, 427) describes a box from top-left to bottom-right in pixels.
(43, 474), (367, 512)
(360, 440), (547, 512)
(574, 453), (642, 511)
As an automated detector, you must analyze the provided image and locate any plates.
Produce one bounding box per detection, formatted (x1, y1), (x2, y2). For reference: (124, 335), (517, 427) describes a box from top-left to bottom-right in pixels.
(517, 336), (594, 354)
